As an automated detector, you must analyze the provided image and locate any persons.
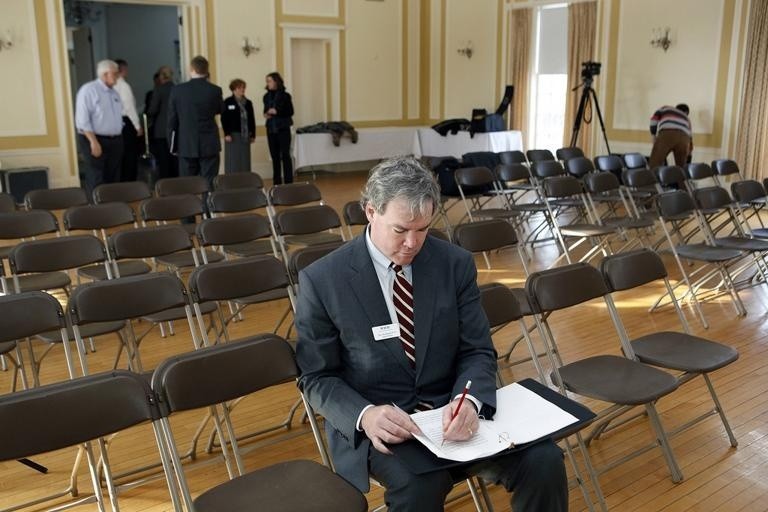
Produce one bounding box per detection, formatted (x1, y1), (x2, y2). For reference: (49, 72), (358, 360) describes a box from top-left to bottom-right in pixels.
(642, 102), (695, 210)
(220, 78), (257, 174)
(148, 65), (179, 178)
(262, 71), (296, 186)
(293, 152), (569, 512)
(111, 58), (144, 181)
(142, 73), (161, 113)
(166, 54), (223, 224)
(74, 58), (126, 205)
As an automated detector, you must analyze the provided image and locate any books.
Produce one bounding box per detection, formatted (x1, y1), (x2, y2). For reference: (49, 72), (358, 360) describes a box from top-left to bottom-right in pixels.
(387, 376), (598, 477)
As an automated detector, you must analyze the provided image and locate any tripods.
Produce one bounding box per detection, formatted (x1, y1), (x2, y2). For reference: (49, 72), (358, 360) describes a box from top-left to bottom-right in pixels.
(569, 80), (611, 156)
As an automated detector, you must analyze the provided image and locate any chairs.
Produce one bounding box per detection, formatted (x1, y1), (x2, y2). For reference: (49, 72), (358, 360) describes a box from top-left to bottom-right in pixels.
(0, 149), (768, 512)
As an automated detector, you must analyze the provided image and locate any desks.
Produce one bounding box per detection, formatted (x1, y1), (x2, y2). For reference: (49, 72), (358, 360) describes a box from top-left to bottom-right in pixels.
(293, 126), (420, 182)
(418, 126), (527, 163)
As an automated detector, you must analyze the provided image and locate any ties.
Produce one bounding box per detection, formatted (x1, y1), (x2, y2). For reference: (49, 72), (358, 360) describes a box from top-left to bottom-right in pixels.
(390, 262), (416, 378)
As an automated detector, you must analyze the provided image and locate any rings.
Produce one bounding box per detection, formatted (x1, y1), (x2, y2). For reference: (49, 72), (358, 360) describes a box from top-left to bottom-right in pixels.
(467, 427), (474, 435)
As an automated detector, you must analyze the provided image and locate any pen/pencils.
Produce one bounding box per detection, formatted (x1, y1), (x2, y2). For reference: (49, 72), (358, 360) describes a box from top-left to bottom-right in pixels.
(441, 380), (472, 446)
